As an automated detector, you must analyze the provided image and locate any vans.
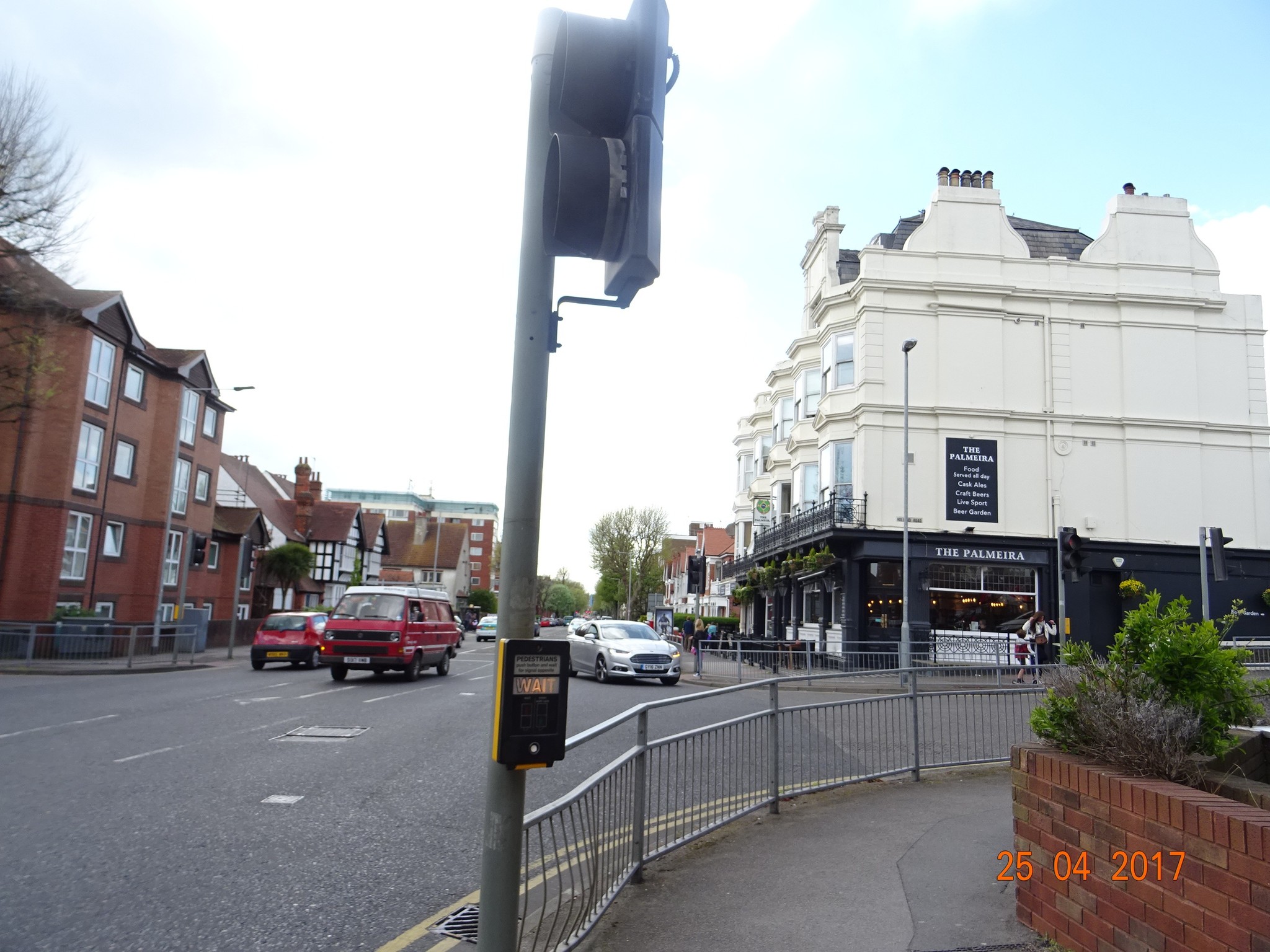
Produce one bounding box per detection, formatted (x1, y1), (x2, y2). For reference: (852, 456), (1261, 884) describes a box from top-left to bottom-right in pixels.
(319, 585), (458, 682)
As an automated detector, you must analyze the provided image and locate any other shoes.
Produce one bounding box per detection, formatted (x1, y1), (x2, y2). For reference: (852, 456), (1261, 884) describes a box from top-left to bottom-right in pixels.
(1032, 679), (1036, 684)
(1017, 678), (1024, 683)
(693, 673), (699, 677)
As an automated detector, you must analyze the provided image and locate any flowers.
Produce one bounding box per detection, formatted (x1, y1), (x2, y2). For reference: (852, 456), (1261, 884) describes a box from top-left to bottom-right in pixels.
(1118, 576), (1146, 601)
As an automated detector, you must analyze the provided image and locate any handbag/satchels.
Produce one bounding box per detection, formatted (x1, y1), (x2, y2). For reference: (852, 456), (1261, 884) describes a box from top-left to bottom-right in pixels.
(690, 646), (696, 655)
(1035, 634), (1048, 644)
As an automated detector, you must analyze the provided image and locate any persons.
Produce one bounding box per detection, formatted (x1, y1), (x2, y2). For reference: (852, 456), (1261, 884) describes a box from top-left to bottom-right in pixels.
(682, 615), (718, 676)
(1014, 610), (1057, 684)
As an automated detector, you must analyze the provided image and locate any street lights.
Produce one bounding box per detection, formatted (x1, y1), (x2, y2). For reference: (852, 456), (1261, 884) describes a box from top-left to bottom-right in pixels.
(616, 551), (632, 621)
(432, 507), (475, 582)
(899, 337), (919, 683)
(610, 578), (620, 620)
(151, 386), (256, 656)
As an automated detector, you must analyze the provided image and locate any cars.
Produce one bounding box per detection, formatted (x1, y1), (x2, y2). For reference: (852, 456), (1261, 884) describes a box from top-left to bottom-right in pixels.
(566, 619), (681, 686)
(534, 622), (540, 637)
(251, 613), (329, 670)
(454, 614), (466, 648)
(534, 611), (612, 628)
(476, 613), (498, 642)
(566, 619), (587, 635)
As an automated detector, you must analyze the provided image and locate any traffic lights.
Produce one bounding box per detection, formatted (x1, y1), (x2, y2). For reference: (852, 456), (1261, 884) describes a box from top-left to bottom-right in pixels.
(688, 555), (707, 595)
(241, 538), (258, 578)
(542, 0), (670, 295)
(189, 533), (207, 566)
(1209, 527), (1236, 581)
(1059, 532), (1083, 581)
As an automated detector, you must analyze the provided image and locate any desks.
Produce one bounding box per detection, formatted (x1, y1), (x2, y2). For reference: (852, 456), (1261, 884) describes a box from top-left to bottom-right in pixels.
(777, 643), (800, 669)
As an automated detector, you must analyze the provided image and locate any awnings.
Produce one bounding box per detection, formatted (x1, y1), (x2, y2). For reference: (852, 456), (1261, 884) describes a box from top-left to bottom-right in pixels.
(294, 575), (324, 594)
(379, 570), (415, 586)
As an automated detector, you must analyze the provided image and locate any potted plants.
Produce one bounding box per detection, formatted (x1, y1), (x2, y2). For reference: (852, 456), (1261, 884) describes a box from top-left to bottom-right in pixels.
(731, 545), (835, 606)
